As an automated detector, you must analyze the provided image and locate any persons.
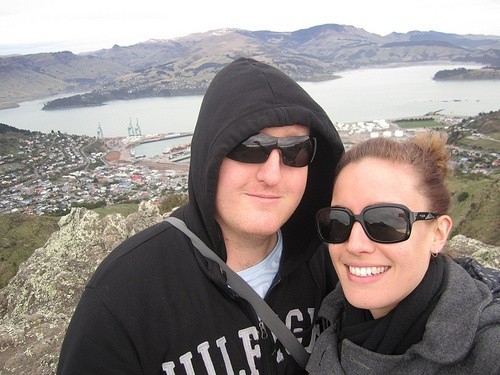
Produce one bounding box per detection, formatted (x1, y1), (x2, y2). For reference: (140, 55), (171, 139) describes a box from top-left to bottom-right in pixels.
(305, 133), (500, 375)
(56, 57), (345, 375)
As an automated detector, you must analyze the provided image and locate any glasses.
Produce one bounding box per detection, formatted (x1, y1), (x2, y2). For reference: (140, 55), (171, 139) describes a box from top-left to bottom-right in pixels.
(315, 202), (442, 244)
(225, 133), (318, 167)
